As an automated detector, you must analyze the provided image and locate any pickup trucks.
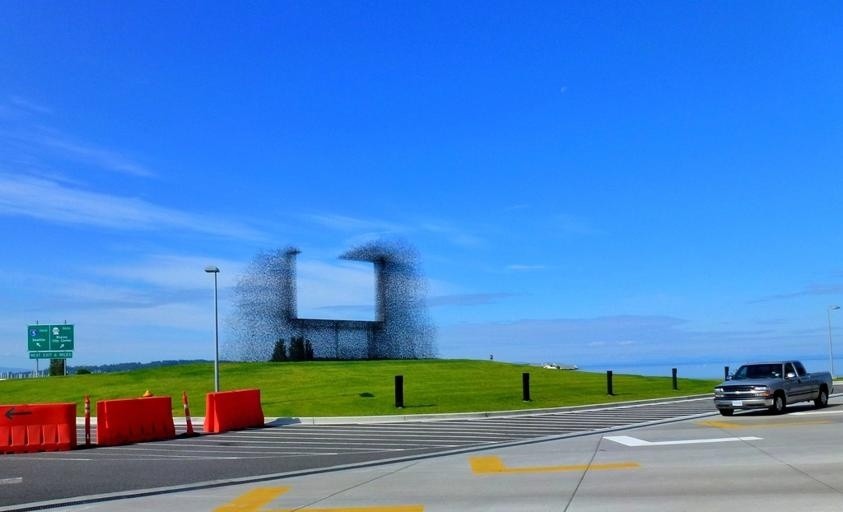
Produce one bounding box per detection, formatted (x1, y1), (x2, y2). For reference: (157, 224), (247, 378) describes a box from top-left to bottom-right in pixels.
(713, 359), (834, 416)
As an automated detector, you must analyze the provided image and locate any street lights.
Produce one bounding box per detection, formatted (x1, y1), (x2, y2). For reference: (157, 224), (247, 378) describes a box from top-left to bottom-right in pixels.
(203, 266), (220, 392)
(827, 305), (840, 378)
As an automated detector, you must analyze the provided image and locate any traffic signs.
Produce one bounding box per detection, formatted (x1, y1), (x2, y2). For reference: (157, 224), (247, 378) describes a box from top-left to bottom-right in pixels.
(28, 325), (75, 359)
